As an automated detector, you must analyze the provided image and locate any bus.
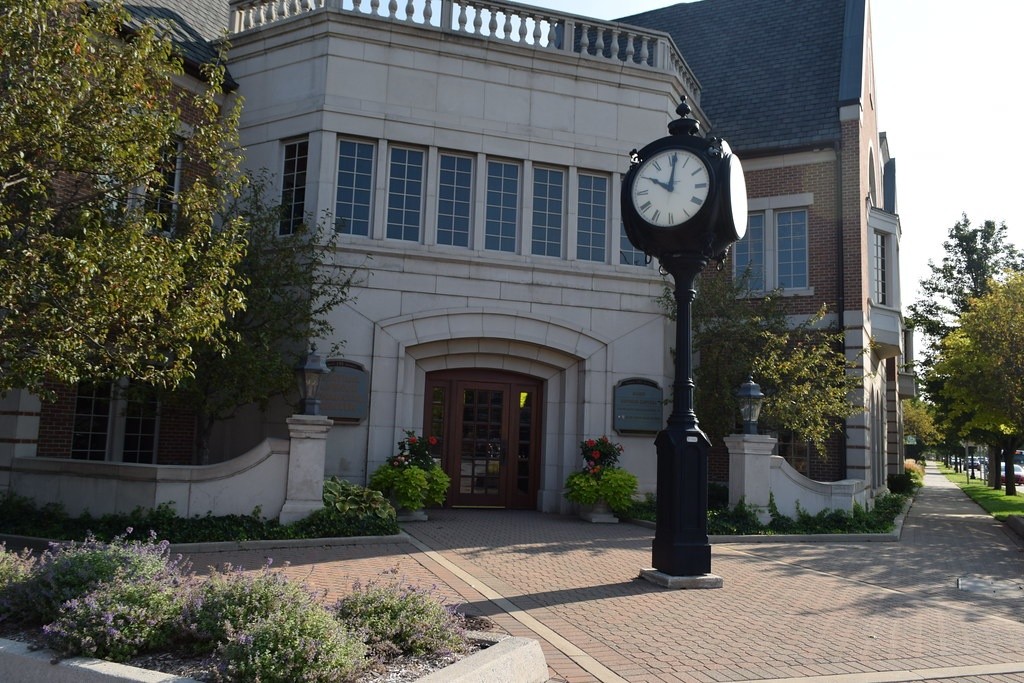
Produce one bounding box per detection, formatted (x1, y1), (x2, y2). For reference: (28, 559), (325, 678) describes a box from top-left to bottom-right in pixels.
(1015, 450), (1024, 468)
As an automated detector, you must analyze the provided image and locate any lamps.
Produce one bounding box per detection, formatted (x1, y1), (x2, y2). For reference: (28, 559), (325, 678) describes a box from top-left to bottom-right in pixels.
(736, 373), (763, 434)
(290, 339), (330, 415)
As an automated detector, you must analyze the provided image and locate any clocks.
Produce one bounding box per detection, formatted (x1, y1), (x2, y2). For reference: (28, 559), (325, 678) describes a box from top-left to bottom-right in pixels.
(631, 147), (709, 226)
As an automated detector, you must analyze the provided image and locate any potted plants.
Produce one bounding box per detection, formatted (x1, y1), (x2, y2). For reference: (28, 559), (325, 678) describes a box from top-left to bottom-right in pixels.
(369, 463), (450, 520)
(562, 467), (637, 522)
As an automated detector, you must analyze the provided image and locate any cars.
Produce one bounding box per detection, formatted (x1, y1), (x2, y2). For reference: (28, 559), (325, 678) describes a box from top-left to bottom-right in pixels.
(950, 458), (960, 465)
(978, 456), (988, 465)
(962, 456), (981, 472)
(1000, 462), (1024, 486)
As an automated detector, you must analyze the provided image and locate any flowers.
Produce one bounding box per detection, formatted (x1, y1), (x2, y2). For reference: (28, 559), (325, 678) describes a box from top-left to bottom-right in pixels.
(580, 435), (624, 474)
(385, 429), (436, 470)
